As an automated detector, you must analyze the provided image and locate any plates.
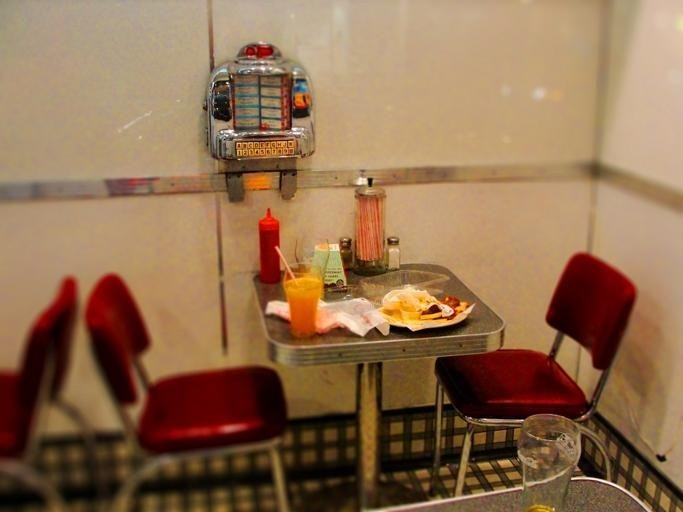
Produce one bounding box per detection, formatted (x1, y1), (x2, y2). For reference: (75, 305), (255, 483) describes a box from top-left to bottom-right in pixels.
(369, 300), (476, 333)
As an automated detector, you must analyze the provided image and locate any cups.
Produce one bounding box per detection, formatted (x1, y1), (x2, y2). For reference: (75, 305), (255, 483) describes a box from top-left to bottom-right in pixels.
(515, 413), (581, 511)
(284, 236), (330, 339)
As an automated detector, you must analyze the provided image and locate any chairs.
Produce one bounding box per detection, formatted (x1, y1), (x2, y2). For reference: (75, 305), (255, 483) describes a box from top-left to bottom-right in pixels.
(430, 252), (638, 498)
(85, 274), (292, 512)
(0, 275), (79, 512)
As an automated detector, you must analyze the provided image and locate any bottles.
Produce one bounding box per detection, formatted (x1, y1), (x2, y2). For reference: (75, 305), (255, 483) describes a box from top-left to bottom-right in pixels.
(338, 178), (400, 272)
(258, 207), (282, 282)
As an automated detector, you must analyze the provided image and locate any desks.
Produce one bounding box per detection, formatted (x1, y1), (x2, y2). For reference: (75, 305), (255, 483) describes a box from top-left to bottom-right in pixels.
(254, 265), (506, 512)
(365, 477), (652, 512)
